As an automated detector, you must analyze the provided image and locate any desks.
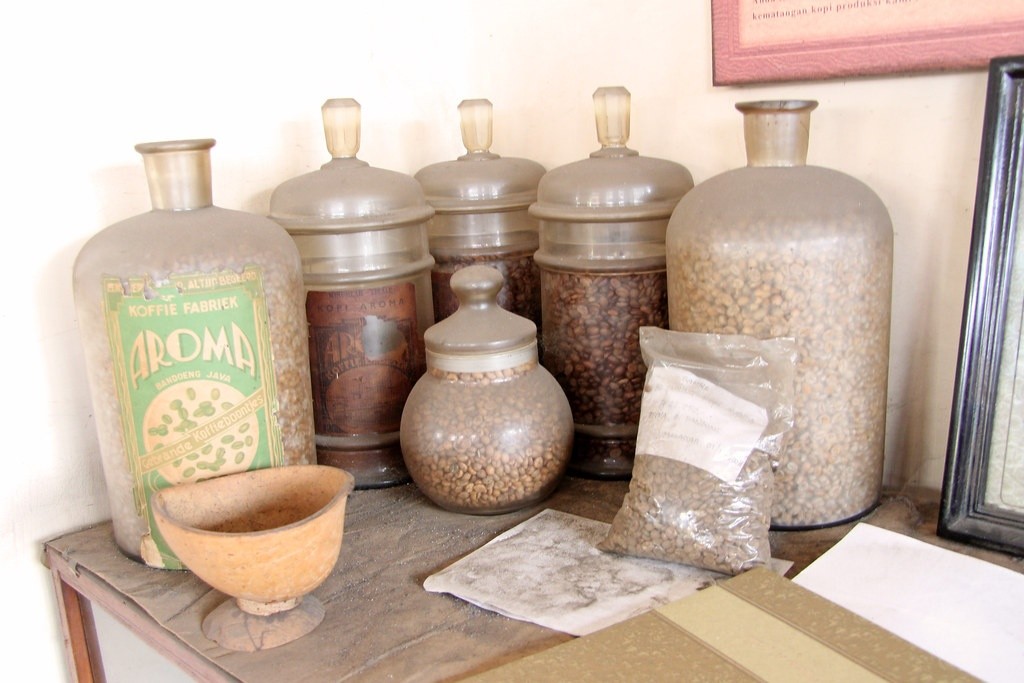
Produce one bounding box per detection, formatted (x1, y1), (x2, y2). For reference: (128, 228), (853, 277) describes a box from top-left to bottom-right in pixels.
(44, 492), (1024, 683)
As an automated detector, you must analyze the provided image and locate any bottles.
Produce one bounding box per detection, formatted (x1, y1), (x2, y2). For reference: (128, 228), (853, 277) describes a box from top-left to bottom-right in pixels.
(665, 100), (894, 530)
(414, 98), (547, 368)
(72, 138), (317, 570)
(268, 99), (436, 490)
(400, 264), (573, 514)
(528, 87), (694, 483)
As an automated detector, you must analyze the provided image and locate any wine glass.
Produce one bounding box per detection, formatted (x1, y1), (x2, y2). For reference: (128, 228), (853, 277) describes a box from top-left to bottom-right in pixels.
(152, 465), (354, 653)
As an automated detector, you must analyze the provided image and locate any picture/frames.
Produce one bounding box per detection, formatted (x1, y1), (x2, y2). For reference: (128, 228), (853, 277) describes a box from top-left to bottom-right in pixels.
(709, 0), (1024, 85)
(936, 56), (1024, 559)
